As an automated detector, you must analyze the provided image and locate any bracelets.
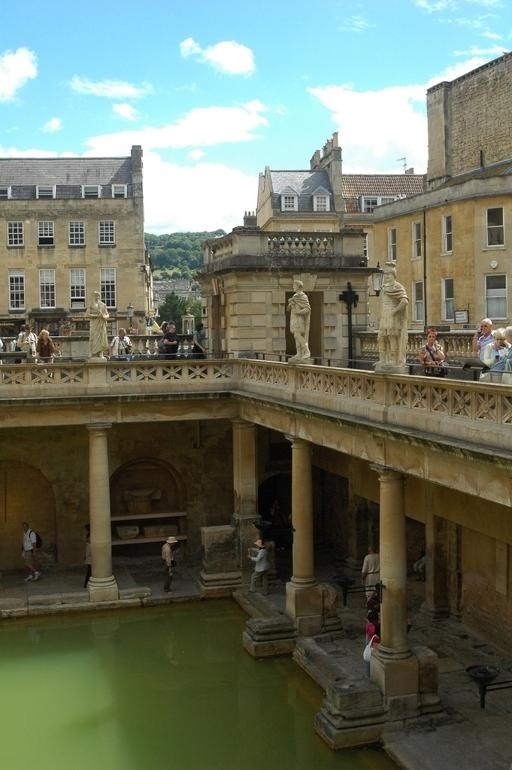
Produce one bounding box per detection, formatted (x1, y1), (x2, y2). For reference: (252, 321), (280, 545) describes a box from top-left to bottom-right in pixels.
(436, 348), (440, 352)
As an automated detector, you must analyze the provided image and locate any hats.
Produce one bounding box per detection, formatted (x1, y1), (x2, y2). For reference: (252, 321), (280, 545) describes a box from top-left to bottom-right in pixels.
(166, 536), (178, 544)
(253, 539), (265, 548)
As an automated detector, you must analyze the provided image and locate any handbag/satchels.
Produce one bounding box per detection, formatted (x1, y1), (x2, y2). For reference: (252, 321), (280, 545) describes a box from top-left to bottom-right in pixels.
(439, 364), (448, 377)
(362, 634), (379, 662)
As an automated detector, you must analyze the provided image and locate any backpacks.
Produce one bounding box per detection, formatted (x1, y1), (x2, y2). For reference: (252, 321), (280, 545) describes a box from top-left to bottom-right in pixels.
(29, 530), (42, 548)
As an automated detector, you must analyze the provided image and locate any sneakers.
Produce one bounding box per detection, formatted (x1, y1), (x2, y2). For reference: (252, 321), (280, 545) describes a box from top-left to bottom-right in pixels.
(31, 571), (40, 581)
(24, 575), (32, 582)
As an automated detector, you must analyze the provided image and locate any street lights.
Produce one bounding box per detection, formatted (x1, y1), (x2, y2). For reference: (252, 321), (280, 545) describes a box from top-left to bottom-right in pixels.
(336, 280), (360, 368)
(126, 302), (136, 334)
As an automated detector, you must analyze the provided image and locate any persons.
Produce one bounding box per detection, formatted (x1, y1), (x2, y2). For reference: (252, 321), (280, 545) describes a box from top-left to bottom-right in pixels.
(82, 524), (91, 588)
(0, 323), (55, 383)
(86, 292), (109, 357)
(373, 262), (409, 366)
(287, 280), (312, 358)
(419, 328), (446, 378)
(471, 318), (512, 384)
(161, 536), (179, 594)
(22, 522), (40, 582)
(360, 544), (425, 680)
(248, 540), (270, 596)
(110, 320), (207, 379)
